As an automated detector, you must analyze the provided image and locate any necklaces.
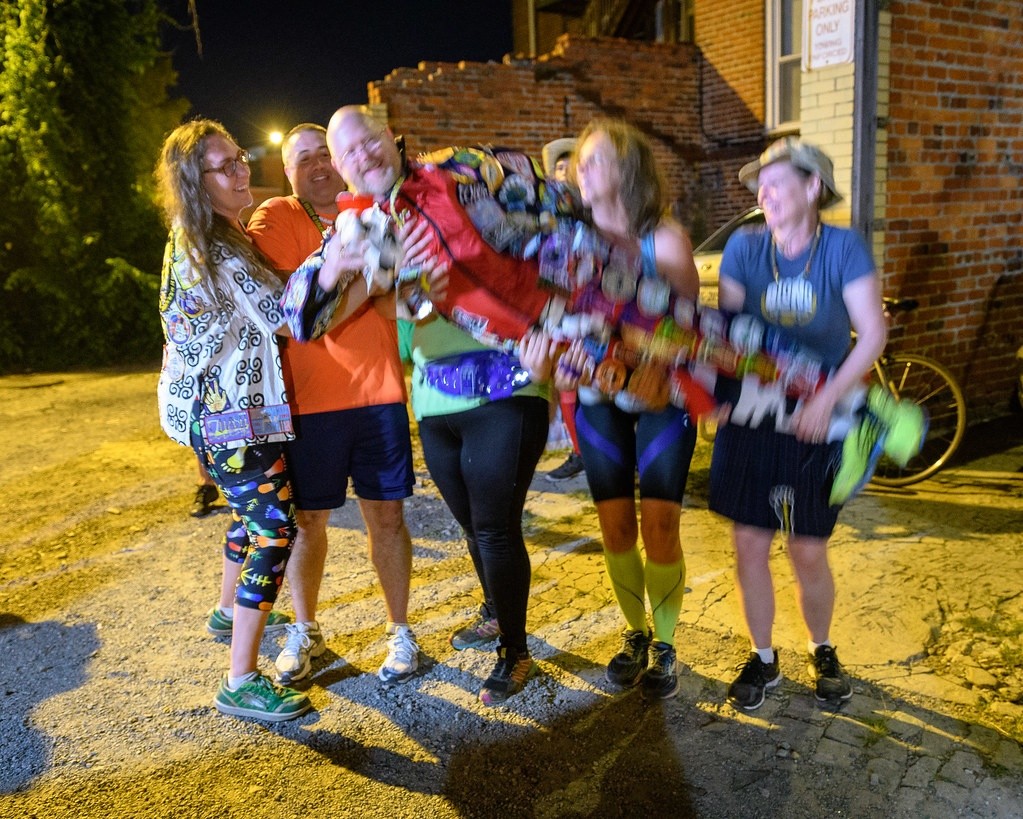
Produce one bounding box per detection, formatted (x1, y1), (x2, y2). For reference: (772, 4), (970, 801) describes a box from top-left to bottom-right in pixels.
(772, 221), (822, 282)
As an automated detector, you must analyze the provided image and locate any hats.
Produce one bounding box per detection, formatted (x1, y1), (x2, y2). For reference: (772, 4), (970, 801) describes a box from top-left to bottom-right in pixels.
(739, 136), (842, 209)
(542, 137), (579, 179)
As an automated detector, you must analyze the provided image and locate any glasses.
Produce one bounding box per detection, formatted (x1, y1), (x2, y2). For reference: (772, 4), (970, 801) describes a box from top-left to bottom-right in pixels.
(201, 149), (249, 176)
(335, 128), (384, 162)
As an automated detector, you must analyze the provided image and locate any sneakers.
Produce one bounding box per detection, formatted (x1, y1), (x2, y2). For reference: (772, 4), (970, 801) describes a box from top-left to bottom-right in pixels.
(806, 644), (853, 700)
(377, 625), (419, 683)
(188, 484), (219, 515)
(205, 607), (291, 634)
(638, 641), (679, 701)
(450, 602), (500, 650)
(478, 645), (536, 706)
(545, 453), (587, 482)
(275, 623), (325, 682)
(604, 624), (652, 687)
(726, 648), (779, 711)
(212, 673), (311, 721)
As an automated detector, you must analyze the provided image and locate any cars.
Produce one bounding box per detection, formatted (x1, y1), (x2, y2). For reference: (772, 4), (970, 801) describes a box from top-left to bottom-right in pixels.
(692, 204), (769, 310)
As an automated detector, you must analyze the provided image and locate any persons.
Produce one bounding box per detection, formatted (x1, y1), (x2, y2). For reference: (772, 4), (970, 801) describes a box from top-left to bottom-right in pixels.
(548, 121), (699, 698)
(704, 137), (888, 715)
(249, 119), (434, 688)
(151, 120), (373, 722)
(277, 99), (595, 707)
(539, 136), (587, 485)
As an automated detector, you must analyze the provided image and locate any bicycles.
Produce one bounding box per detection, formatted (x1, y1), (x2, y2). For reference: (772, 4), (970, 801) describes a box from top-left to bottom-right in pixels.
(848, 293), (970, 488)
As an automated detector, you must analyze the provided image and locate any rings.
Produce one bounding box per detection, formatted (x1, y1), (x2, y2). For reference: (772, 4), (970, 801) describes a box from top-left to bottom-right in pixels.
(341, 247), (347, 257)
(410, 258), (414, 266)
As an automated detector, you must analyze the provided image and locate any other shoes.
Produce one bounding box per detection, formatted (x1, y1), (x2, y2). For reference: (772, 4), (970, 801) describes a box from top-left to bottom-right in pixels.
(831, 412), (885, 511)
(872, 396), (925, 464)
